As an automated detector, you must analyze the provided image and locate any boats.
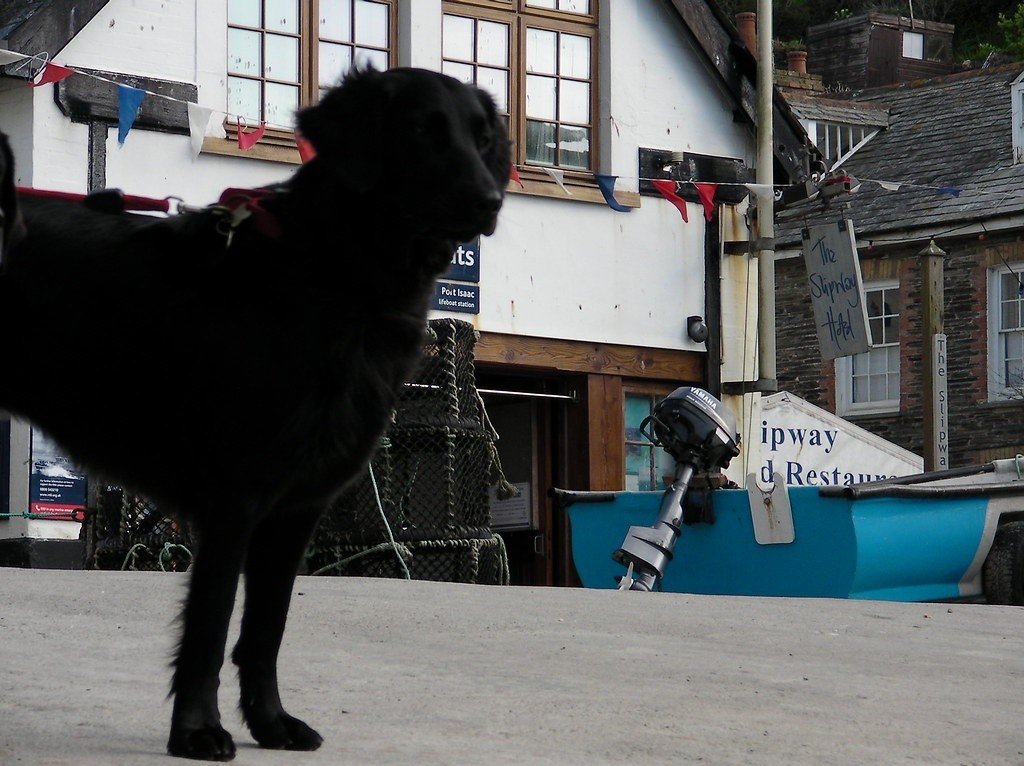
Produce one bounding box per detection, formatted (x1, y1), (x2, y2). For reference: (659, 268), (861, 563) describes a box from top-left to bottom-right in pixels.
(564, 387), (1024, 609)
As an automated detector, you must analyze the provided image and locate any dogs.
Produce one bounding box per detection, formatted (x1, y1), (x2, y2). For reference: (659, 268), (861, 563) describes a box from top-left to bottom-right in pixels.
(1, 57), (516, 762)
